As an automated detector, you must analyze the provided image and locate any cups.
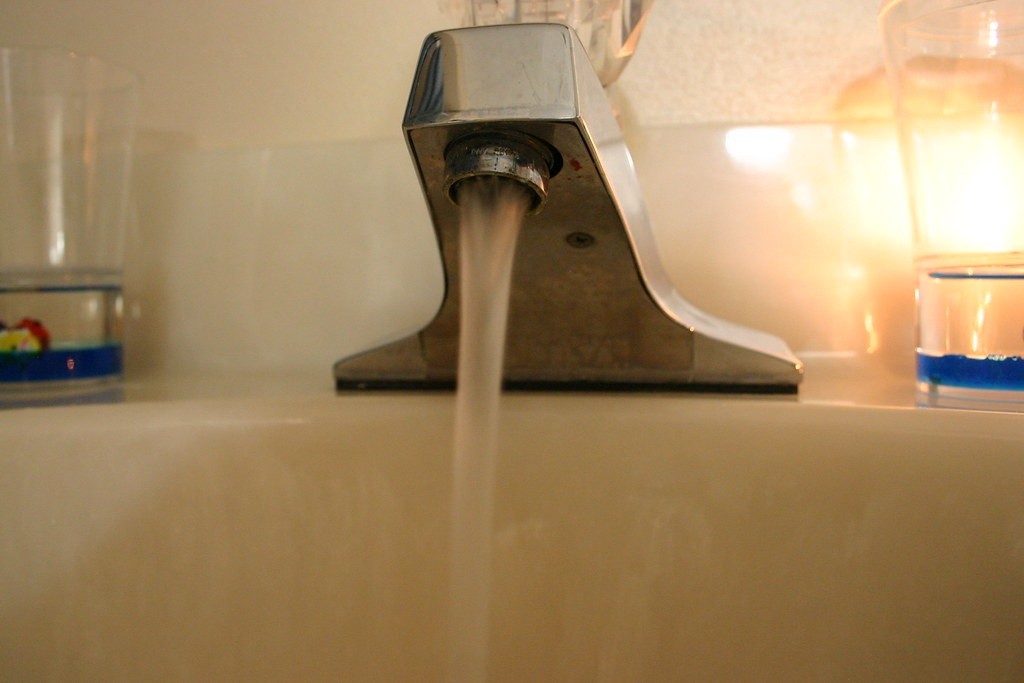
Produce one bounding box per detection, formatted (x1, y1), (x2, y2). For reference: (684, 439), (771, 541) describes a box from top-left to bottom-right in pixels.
(880, 0), (1024, 412)
(0, 45), (139, 404)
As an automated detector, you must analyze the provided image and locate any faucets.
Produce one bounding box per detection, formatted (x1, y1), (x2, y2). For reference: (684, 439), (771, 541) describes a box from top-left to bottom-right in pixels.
(331, 22), (805, 395)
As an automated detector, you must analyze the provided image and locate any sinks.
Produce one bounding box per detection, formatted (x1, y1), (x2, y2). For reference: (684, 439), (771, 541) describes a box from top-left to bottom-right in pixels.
(0, 392), (1024, 683)
